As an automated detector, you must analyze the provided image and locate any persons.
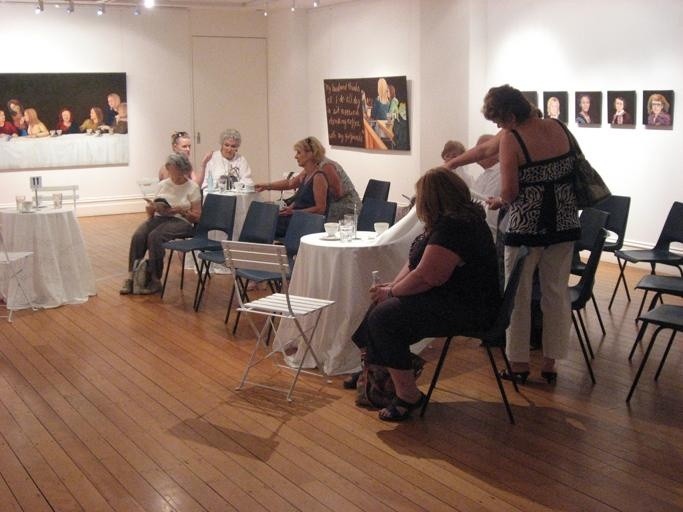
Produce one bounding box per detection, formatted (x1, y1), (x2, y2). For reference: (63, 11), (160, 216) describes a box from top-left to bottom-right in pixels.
(53, 105), (82, 134)
(344, 76), (614, 425)
(105, 93), (121, 125)
(7, 98), (27, 136)
(200, 127), (255, 191)
(157, 132), (214, 264)
(20, 108), (49, 138)
(119, 150), (203, 294)
(112, 101), (128, 134)
(78, 106), (106, 132)
(608, 96), (635, 125)
(242, 137), (330, 291)
(306, 135), (363, 225)
(646, 93), (671, 127)
(0, 110), (19, 137)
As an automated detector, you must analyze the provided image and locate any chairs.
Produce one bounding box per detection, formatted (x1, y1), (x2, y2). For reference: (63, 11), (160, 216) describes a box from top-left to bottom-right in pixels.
(0, 230), (38, 323)
(33, 186), (79, 214)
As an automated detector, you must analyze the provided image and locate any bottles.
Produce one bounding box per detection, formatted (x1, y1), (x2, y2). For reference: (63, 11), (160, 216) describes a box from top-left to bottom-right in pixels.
(279, 201), (288, 211)
(207, 175), (213, 192)
(372, 271), (382, 287)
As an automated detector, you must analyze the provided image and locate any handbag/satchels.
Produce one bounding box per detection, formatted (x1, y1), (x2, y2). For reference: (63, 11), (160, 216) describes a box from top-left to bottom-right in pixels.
(132, 258), (150, 294)
(573, 148), (612, 210)
(356, 346), (427, 406)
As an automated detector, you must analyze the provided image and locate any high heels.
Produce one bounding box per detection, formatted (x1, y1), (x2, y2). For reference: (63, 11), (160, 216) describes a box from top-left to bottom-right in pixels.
(542, 371), (557, 384)
(499, 369), (530, 383)
(344, 371), (361, 390)
(379, 390), (426, 421)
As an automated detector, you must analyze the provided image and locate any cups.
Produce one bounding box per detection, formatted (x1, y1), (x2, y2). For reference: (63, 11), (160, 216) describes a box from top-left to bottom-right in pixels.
(50, 130), (56, 136)
(234, 182), (243, 190)
(57, 130), (62, 136)
(87, 129), (92, 134)
(15, 193), (63, 213)
(246, 185), (255, 191)
(323, 213), (390, 241)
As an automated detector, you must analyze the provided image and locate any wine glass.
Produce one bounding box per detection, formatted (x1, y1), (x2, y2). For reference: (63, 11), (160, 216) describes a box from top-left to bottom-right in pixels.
(29, 176), (44, 208)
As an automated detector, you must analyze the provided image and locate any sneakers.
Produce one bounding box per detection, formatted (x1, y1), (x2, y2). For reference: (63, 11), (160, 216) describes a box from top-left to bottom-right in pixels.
(141, 280), (161, 293)
(120, 279), (131, 293)
